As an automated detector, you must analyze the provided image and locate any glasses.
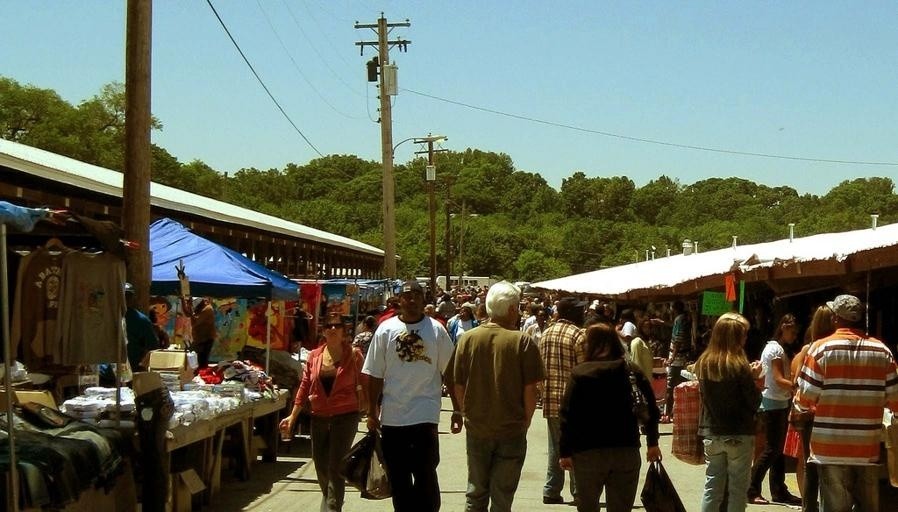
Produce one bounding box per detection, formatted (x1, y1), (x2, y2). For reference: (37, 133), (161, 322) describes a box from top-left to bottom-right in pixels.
(323, 323), (343, 329)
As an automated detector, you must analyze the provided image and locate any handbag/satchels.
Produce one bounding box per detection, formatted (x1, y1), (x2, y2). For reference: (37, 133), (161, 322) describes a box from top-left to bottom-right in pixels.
(631, 386), (649, 426)
(354, 382), (368, 415)
(791, 403), (813, 431)
(13, 402), (74, 429)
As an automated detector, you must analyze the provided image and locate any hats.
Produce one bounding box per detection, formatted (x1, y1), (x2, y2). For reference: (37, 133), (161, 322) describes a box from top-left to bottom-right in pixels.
(826, 295), (863, 322)
(124, 283), (135, 291)
(557, 297), (587, 312)
(399, 282), (423, 299)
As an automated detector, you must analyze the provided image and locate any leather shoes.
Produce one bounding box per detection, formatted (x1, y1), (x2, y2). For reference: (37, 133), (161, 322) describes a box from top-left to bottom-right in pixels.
(672, 380), (705, 464)
(772, 489), (797, 502)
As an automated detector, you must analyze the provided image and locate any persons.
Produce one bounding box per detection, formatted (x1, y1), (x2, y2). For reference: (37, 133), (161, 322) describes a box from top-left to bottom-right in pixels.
(787, 294), (896, 512)
(694, 312), (765, 512)
(122, 282), (161, 372)
(360, 281), (463, 512)
(539, 297), (588, 505)
(279, 318), (368, 510)
(557, 323), (662, 512)
(148, 306), (170, 348)
(130, 371), (177, 512)
(749, 314), (804, 506)
(180, 295), (217, 377)
(352, 280), (693, 424)
(454, 280), (544, 511)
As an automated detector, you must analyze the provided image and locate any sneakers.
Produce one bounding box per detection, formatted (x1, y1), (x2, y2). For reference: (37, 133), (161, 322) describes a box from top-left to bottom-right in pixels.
(543, 497), (563, 504)
(661, 415), (670, 424)
(750, 495), (769, 504)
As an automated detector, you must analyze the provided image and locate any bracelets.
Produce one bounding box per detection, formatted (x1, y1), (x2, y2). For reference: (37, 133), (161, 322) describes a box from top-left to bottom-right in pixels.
(191, 313), (195, 316)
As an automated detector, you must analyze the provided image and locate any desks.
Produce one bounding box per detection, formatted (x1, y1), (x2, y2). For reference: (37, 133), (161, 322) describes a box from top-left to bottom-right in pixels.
(39, 389), (289, 512)
